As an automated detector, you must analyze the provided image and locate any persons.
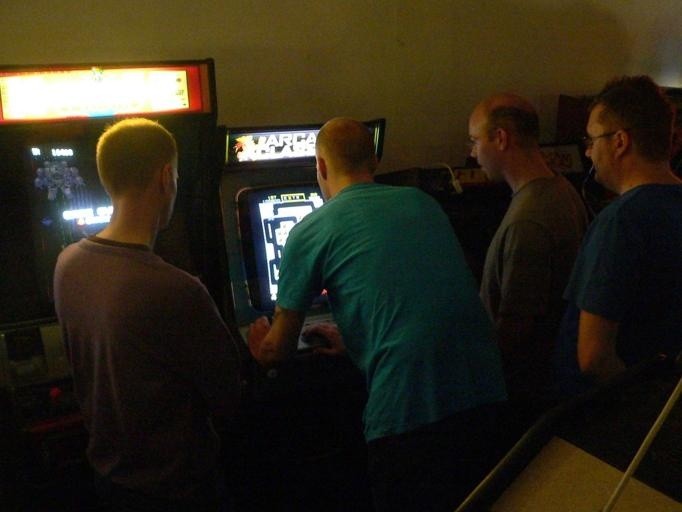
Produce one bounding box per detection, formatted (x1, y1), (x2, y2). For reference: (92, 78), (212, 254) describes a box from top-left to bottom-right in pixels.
(52, 117), (243, 512)
(556, 73), (682, 501)
(247, 116), (512, 510)
(466, 92), (589, 460)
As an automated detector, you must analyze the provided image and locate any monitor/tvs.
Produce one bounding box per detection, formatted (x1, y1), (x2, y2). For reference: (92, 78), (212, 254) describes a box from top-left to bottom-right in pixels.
(245, 180), (331, 309)
(27, 165), (195, 311)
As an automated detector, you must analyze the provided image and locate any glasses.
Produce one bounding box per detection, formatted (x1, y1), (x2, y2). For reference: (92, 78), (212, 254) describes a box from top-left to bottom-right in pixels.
(580, 126), (625, 149)
